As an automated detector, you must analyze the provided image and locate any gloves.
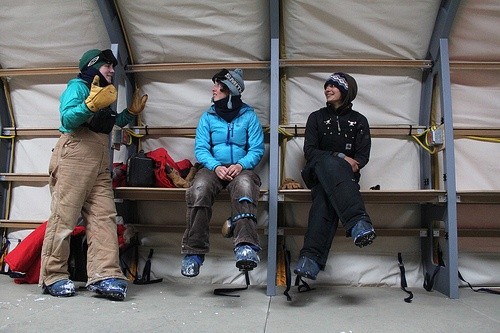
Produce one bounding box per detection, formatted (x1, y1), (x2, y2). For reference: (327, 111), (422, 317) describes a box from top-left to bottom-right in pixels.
(85, 75), (118, 113)
(281, 176), (303, 190)
(127, 88), (148, 115)
(166, 165), (196, 188)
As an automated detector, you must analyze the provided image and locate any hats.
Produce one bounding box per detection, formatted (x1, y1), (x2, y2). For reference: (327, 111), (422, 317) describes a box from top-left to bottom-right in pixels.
(212, 68), (245, 109)
(78, 48), (118, 70)
(324, 73), (348, 95)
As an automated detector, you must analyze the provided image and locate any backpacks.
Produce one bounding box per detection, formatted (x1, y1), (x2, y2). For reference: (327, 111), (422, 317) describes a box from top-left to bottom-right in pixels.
(144, 147), (193, 189)
(112, 161), (126, 187)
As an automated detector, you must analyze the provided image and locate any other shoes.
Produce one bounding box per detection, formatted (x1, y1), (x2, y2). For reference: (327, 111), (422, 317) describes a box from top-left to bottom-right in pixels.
(43, 279), (75, 297)
(293, 256), (320, 280)
(180, 253), (205, 277)
(233, 245), (260, 269)
(87, 278), (129, 301)
(351, 219), (377, 248)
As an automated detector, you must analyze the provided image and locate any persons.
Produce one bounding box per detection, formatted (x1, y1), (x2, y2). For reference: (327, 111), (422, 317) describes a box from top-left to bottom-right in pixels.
(294, 72), (376, 280)
(180, 68), (266, 276)
(37, 50), (147, 301)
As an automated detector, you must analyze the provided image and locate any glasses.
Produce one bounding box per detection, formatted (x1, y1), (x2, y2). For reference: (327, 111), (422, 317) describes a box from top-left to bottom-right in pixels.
(80, 49), (118, 73)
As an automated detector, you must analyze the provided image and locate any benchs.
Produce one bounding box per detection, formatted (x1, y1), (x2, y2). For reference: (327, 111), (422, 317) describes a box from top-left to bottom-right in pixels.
(112, 182), (500, 239)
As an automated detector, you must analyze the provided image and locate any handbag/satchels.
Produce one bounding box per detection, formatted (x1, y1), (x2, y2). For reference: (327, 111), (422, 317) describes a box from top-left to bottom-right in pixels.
(125, 156), (156, 187)
